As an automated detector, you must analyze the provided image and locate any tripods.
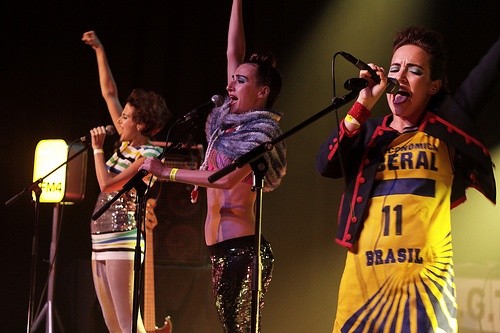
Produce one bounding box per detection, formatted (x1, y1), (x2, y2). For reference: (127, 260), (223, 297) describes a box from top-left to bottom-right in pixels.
(29, 205), (64, 333)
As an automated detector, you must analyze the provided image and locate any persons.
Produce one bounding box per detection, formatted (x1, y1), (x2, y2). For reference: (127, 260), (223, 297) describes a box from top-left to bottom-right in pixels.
(83, 31), (168, 333)
(138, 0), (288, 333)
(317, 25), (500, 333)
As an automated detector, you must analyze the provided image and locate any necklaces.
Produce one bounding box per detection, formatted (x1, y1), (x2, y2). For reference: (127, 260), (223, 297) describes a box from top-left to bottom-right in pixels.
(190, 127), (225, 203)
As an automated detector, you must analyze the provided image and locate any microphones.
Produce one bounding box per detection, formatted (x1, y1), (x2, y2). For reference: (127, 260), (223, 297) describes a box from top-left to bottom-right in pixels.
(74, 125), (115, 143)
(176, 95), (224, 124)
(340, 51), (400, 94)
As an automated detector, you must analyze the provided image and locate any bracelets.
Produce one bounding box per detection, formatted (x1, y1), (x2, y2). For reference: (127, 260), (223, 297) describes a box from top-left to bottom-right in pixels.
(344, 114), (361, 125)
(94, 149), (104, 154)
(170, 168), (178, 182)
